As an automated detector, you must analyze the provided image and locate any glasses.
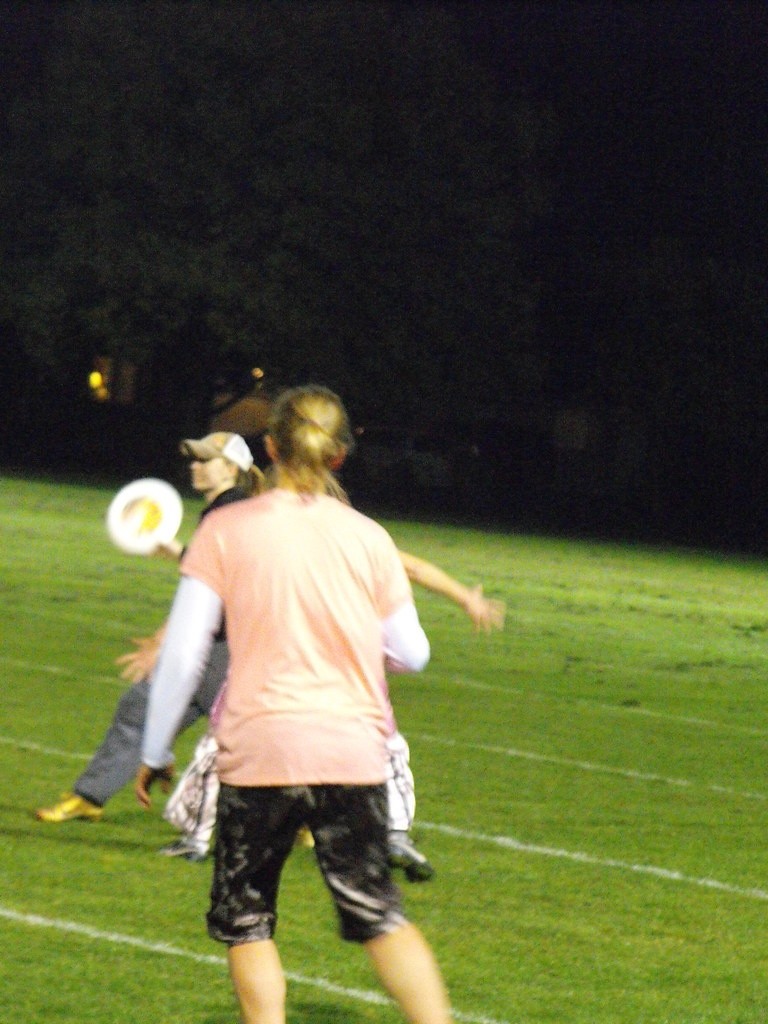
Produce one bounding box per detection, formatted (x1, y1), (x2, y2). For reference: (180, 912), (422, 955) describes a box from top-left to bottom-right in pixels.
(193, 455), (213, 463)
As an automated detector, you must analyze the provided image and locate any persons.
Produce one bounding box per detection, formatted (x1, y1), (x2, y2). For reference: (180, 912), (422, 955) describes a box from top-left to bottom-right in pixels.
(37, 399), (505, 1024)
(136, 387), (455, 1024)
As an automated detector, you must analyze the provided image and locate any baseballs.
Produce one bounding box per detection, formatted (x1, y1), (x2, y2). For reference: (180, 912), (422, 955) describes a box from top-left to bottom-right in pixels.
(89, 372), (108, 399)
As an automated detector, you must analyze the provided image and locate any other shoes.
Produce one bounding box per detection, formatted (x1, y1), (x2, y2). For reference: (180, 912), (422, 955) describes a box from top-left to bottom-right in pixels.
(35, 792), (103, 823)
(294, 827), (314, 848)
(386, 840), (432, 880)
(154, 839), (209, 863)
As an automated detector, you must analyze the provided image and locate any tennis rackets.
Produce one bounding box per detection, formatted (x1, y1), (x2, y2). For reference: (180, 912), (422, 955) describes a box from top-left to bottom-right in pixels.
(104, 476), (192, 564)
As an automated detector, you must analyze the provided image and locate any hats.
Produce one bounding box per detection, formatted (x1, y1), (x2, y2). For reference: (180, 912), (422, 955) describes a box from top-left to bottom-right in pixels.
(179, 432), (253, 472)
